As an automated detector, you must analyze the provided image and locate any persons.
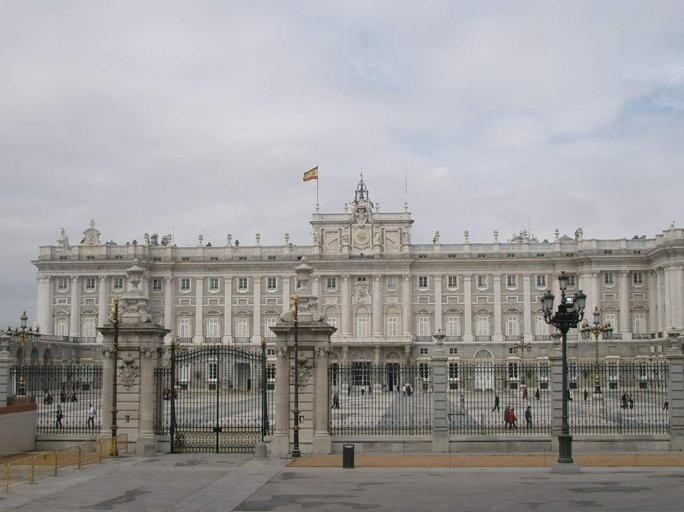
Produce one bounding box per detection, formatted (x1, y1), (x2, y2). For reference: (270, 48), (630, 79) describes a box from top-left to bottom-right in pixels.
(88, 404), (94, 429)
(332, 383), (413, 409)
(584, 390), (587, 401)
(622, 391), (632, 409)
(44, 384), (78, 405)
(492, 386), (539, 430)
(567, 387), (573, 400)
(56, 405), (63, 429)
(663, 394), (668, 410)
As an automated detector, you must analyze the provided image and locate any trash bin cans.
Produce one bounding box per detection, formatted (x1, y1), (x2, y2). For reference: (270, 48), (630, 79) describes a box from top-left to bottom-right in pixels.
(343, 444), (354, 467)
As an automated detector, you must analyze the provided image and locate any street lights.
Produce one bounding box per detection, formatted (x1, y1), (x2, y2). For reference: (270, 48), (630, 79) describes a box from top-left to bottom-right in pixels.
(109, 295), (119, 456)
(291, 294), (300, 457)
(513, 334), (531, 384)
(7, 312), (41, 395)
(540, 271), (586, 463)
(580, 306), (613, 393)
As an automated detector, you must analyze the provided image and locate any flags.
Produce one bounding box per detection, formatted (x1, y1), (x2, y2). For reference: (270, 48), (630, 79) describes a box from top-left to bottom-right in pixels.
(303, 166), (318, 181)
